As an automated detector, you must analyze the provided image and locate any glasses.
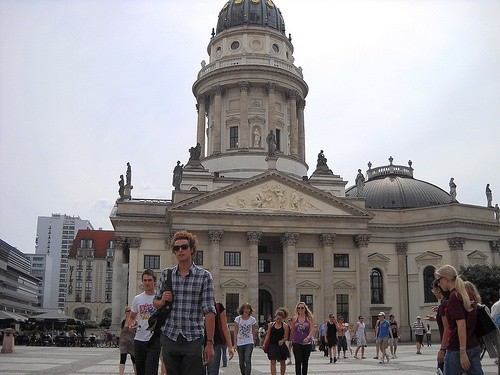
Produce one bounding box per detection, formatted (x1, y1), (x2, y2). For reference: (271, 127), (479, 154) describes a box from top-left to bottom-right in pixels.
(330, 317), (334, 318)
(359, 318), (363, 319)
(297, 306), (306, 309)
(174, 244), (190, 251)
(126, 310), (131, 313)
(431, 289), (438, 292)
(436, 277), (443, 283)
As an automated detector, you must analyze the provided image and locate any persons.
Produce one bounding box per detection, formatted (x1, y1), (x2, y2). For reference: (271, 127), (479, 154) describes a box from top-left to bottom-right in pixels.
(28, 326), (118, 348)
(233, 303), (258, 375)
(423, 265), (500, 375)
(486, 184), (493, 208)
(189, 143), (203, 160)
(388, 313), (400, 358)
(119, 306), (138, 375)
(153, 230), (217, 375)
(258, 302), (367, 375)
(317, 149), (329, 170)
(373, 312), (394, 363)
(203, 301), (234, 375)
(173, 161), (183, 190)
(425, 324), (432, 348)
(355, 169), (365, 197)
(411, 315), (425, 354)
(130, 268), (161, 375)
(119, 162), (131, 201)
(449, 178), (457, 202)
(254, 128), (261, 147)
(266, 130), (277, 157)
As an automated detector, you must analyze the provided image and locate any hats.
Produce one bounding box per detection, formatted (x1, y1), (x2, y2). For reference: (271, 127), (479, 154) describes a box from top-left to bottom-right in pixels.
(339, 316), (344, 320)
(417, 316), (421, 318)
(378, 311), (385, 316)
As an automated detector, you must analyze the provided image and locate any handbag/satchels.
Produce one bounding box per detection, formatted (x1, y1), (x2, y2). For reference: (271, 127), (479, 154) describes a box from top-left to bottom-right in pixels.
(467, 288), (500, 337)
(216, 301), (230, 349)
(146, 268), (173, 333)
(421, 322), (427, 335)
(263, 322), (274, 353)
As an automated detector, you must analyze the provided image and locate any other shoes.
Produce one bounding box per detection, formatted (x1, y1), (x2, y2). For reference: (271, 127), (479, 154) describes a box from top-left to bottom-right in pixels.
(330, 354), (398, 364)
(286, 360), (296, 365)
(416, 352), (421, 354)
